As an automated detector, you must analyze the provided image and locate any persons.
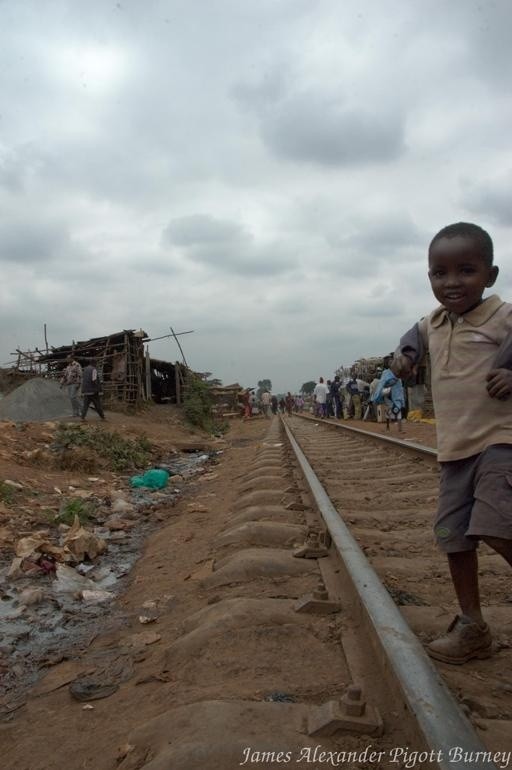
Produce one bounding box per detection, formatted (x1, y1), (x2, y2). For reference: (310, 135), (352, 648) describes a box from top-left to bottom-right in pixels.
(387, 220), (511, 665)
(79, 359), (109, 421)
(233, 350), (429, 434)
(59, 354), (84, 417)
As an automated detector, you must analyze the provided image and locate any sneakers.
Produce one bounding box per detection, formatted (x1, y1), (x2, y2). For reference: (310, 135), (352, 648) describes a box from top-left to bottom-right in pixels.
(425, 613), (493, 665)
